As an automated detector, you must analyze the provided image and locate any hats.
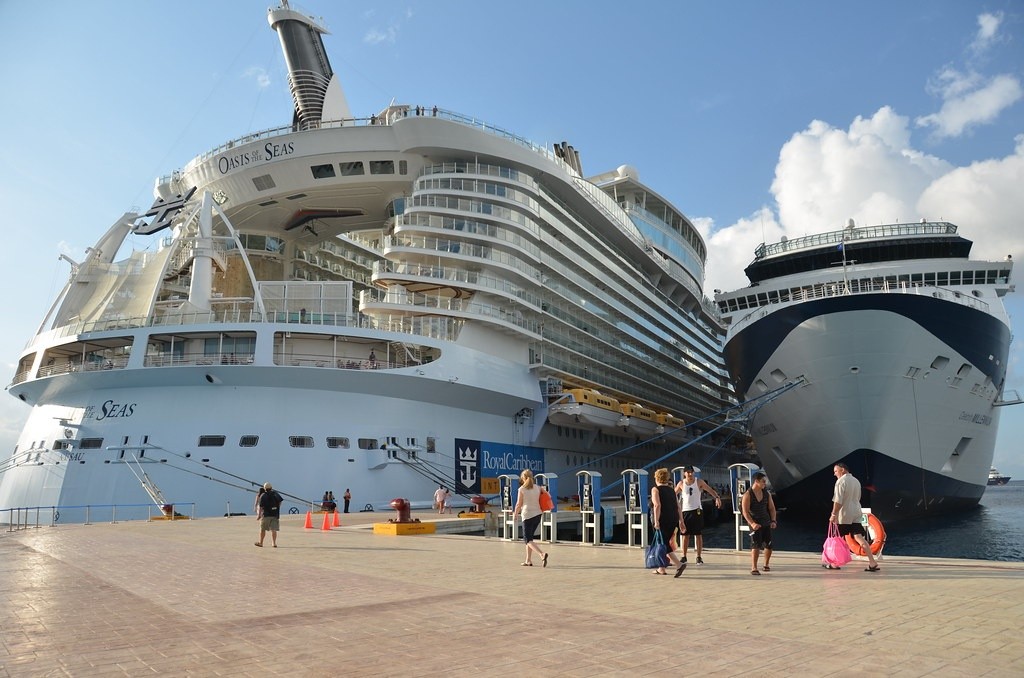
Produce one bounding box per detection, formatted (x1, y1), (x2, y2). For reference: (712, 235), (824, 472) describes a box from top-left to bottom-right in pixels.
(264, 482), (271, 490)
(684, 465), (694, 471)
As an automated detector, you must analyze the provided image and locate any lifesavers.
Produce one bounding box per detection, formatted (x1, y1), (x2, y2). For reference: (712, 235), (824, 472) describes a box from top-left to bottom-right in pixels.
(844, 512), (885, 556)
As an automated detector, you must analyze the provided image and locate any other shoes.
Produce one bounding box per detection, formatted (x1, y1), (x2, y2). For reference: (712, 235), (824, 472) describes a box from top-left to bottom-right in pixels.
(255, 542), (263, 547)
(439, 511), (444, 514)
(680, 557), (687, 563)
(751, 570), (761, 575)
(273, 545), (277, 547)
(257, 516), (261, 520)
(696, 557), (703, 565)
(762, 566), (770, 571)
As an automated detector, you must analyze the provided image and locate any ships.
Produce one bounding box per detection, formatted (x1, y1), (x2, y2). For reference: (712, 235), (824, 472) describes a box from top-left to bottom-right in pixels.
(0, 0), (763, 527)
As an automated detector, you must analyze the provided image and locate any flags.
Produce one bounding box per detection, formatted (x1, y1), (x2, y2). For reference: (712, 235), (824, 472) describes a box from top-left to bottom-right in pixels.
(835, 244), (843, 251)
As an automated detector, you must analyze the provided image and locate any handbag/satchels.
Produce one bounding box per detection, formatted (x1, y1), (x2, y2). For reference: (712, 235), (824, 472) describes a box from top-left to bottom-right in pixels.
(432, 503), (438, 510)
(645, 529), (668, 569)
(539, 487), (554, 512)
(821, 522), (853, 567)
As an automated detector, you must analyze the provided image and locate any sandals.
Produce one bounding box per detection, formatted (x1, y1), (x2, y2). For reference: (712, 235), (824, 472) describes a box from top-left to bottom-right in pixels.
(865, 564), (880, 571)
(825, 564), (840, 570)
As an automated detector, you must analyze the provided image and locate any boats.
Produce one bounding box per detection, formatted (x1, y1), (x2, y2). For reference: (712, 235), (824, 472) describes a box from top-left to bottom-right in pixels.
(656, 411), (691, 437)
(556, 386), (623, 422)
(713, 217), (1024, 529)
(619, 401), (663, 435)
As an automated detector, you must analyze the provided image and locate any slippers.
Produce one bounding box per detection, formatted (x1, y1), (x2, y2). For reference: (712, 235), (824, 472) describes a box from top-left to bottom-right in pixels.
(652, 570), (667, 575)
(520, 562), (532, 566)
(674, 564), (686, 578)
(542, 553), (548, 567)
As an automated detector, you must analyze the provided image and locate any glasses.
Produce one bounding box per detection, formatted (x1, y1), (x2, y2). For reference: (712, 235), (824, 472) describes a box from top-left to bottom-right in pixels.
(689, 487), (693, 495)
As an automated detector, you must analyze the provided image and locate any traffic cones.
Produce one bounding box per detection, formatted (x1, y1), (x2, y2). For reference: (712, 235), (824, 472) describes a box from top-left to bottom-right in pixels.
(330, 509), (342, 527)
(320, 511), (333, 530)
(303, 510), (314, 529)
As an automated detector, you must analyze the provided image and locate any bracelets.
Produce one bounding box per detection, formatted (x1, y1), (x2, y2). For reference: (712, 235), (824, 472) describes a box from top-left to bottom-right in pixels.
(771, 520), (776, 524)
(716, 496), (720, 499)
(831, 512), (837, 517)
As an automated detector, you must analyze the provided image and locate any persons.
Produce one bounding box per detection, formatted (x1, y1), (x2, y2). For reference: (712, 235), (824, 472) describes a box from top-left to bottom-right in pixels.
(673, 466), (721, 565)
(442, 488), (454, 514)
(824, 464), (883, 571)
(371, 114), (376, 125)
(364, 352), (375, 367)
(336, 360), (362, 370)
(434, 485), (446, 514)
(322, 490), (337, 510)
(416, 104), (425, 116)
(651, 469), (687, 578)
(222, 355), (229, 365)
(344, 489), (351, 513)
(300, 308), (306, 322)
(432, 105), (438, 117)
(741, 472), (777, 575)
(254, 488), (266, 517)
(399, 108), (408, 117)
(230, 353), (238, 365)
(254, 482), (284, 547)
(513, 469), (552, 568)
